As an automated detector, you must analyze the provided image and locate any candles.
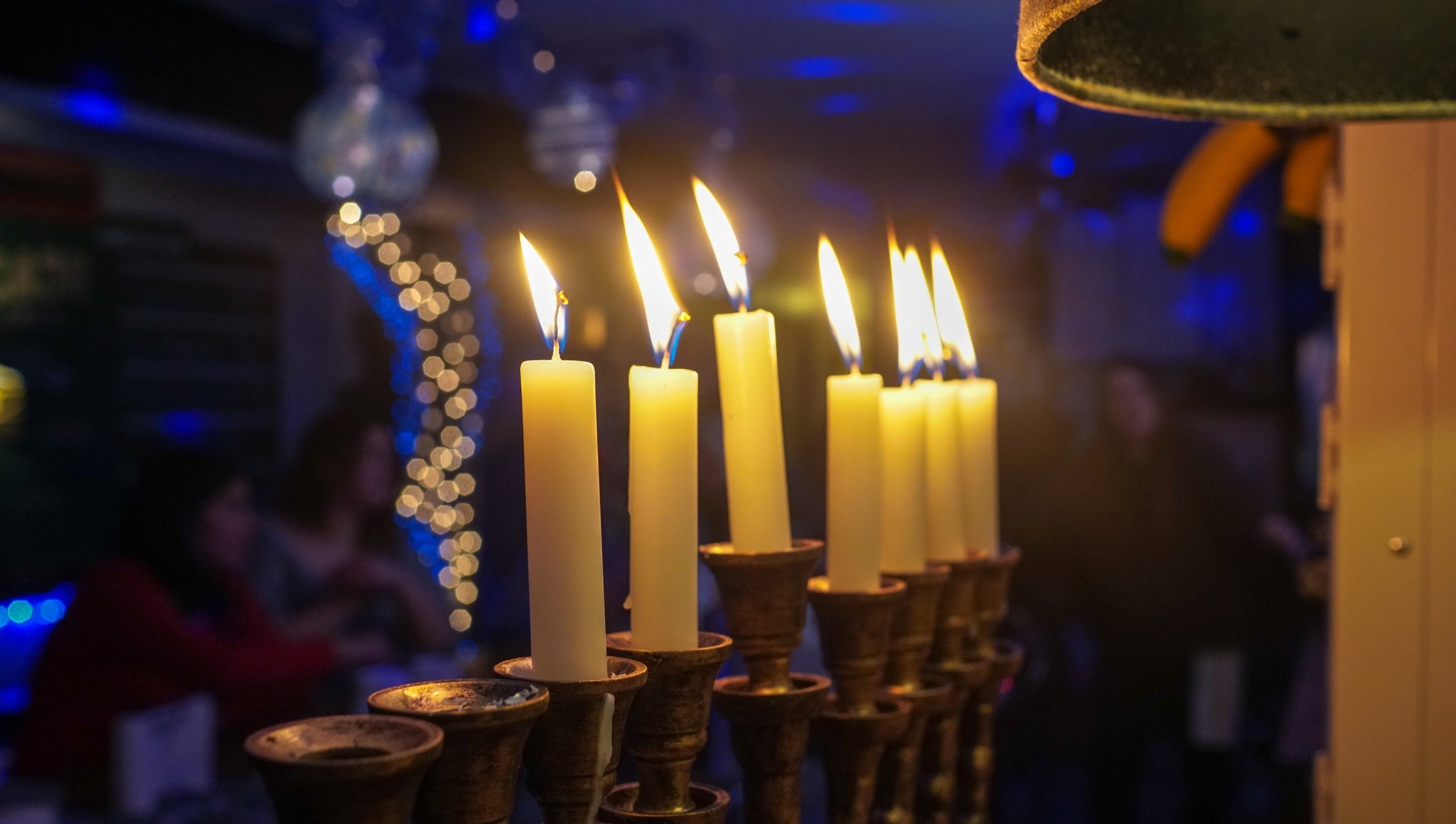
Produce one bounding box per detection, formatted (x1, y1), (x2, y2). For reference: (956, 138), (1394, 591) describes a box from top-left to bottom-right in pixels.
(611, 170), (697, 650)
(517, 229), (608, 680)
(689, 175), (791, 555)
(818, 224), (999, 592)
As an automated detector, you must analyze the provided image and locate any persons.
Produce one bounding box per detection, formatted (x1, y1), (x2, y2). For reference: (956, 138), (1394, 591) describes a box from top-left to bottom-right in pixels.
(27, 381), (456, 824)
(1070, 356), (1329, 824)
